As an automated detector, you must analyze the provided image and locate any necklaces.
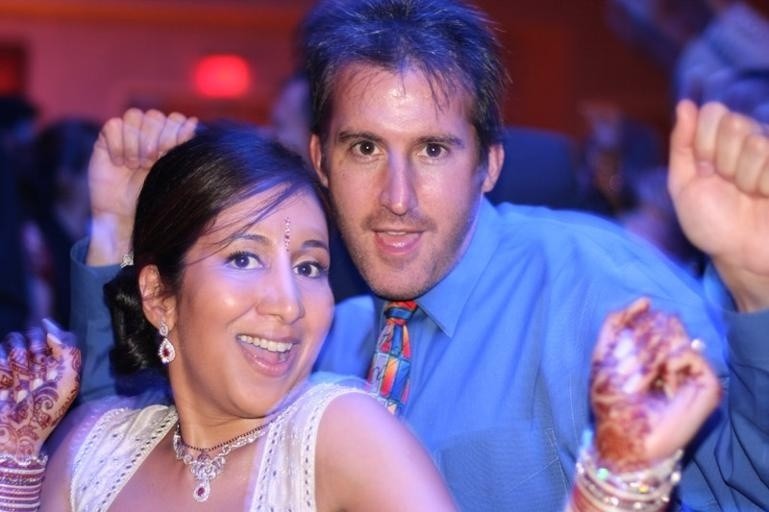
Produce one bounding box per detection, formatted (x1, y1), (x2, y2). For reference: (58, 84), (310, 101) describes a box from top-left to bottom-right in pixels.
(168, 416), (278, 500)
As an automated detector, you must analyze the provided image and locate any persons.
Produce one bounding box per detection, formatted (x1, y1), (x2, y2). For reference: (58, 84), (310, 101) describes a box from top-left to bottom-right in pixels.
(16, 117), (106, 331)
(59, 0), (769, 511)
(0, 129), (722, 511)
(2, 42), (46, 329)
(276, 72), (326, 183)
(606, 2), (769, 141)
(584, 107), (695, 262)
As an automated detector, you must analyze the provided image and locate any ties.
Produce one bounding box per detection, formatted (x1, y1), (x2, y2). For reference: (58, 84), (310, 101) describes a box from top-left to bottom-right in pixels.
(360, 301), (420, 417)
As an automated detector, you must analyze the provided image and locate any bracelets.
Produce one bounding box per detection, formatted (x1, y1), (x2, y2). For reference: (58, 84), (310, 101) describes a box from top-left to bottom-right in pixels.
(572, 428), (687, 511)
(0, 454), (51, 510)
